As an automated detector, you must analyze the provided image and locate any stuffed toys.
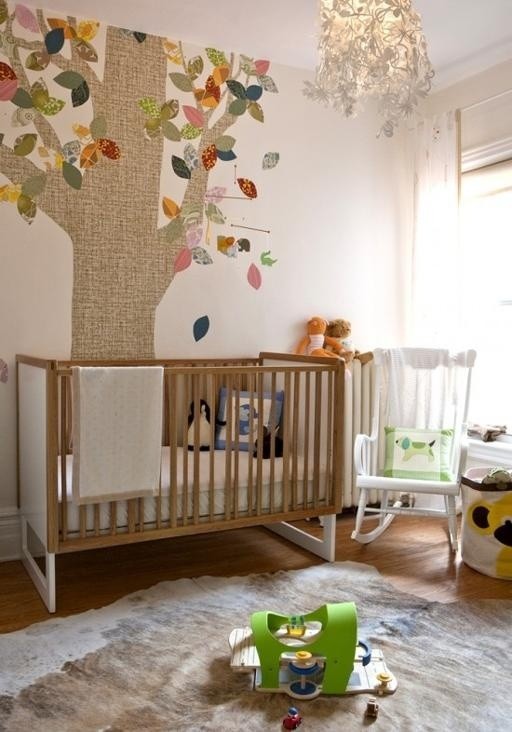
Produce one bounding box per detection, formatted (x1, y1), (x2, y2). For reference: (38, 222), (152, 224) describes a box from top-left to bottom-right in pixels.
(324, 317), (373, 366)
(186, 400), (226, 451)
(296, 316), (345, 364)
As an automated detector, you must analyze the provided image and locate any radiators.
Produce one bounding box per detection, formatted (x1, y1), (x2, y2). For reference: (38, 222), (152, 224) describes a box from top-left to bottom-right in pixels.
(306, 349), (400, 530)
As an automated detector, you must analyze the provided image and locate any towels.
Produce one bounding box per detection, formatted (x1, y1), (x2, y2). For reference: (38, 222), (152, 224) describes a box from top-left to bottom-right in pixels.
(70, 365), (165, 508)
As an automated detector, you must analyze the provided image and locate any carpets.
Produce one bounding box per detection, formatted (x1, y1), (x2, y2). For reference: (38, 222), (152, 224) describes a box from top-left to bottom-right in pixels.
(0, 559), (512, 732)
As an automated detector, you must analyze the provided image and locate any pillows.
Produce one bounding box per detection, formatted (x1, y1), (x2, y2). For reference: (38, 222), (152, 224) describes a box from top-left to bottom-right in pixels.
(213, 386), (287, 455)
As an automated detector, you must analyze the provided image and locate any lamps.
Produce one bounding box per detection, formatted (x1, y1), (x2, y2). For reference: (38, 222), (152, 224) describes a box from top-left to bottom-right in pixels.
(300, 0), (437, 139)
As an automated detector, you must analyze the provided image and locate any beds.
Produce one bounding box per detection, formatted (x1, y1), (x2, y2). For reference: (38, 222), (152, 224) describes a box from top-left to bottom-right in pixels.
(15, 351), (346, 614)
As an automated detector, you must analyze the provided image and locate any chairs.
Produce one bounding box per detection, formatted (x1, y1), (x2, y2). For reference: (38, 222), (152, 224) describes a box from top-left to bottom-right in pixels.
(350, 346), (476, 552)
